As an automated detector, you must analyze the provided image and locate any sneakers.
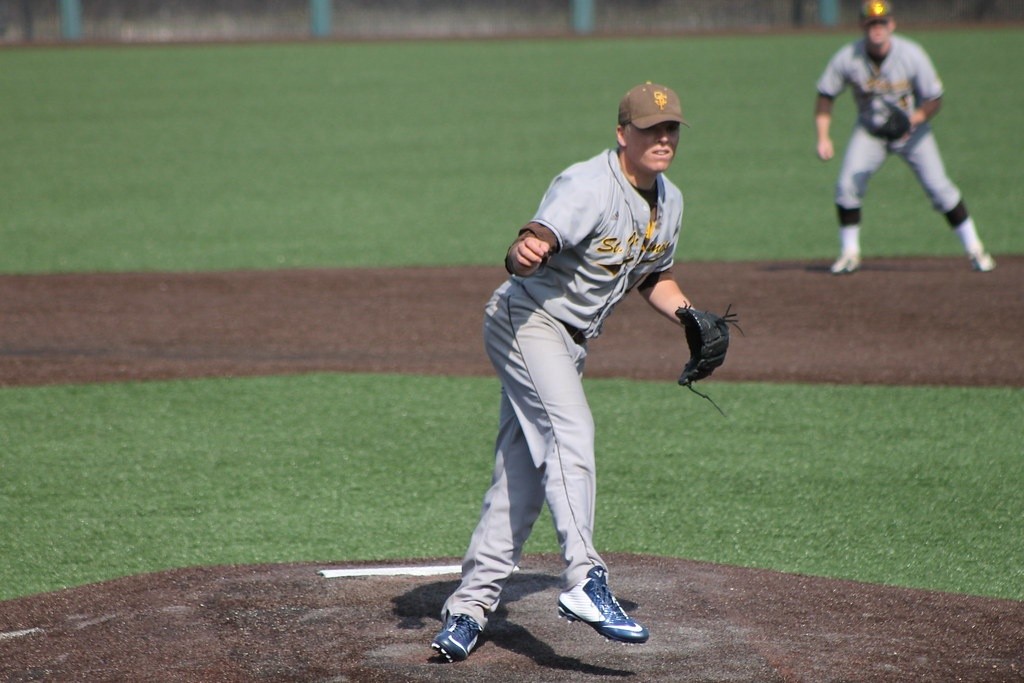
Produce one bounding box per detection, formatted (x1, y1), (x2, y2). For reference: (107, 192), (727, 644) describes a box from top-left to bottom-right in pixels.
(430, 611), (482, 663)
(558, 564), (649, 647)
(831, 250), (861, 272)
(971, 253), (997, 271)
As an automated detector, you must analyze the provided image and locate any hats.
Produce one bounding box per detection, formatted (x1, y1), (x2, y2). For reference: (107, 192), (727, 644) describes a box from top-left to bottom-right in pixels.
(618, 81), (693, 130)
(859, 0), (894, 27)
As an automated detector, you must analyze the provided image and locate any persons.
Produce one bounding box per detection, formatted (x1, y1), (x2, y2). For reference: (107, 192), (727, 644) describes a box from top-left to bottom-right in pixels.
(423, 79), (733, 664)
(810, 0), (996, 279)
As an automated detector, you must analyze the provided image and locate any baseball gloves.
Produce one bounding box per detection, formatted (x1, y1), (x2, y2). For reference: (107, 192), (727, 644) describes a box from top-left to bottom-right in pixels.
(675, 301), (744, 418)
